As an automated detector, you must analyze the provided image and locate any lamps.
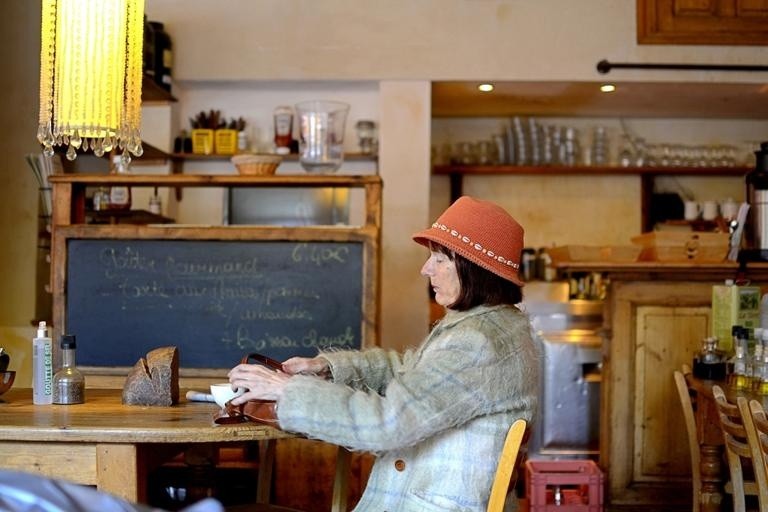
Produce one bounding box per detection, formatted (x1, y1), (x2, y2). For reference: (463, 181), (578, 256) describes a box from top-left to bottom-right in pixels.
(36, 0), (146, 166)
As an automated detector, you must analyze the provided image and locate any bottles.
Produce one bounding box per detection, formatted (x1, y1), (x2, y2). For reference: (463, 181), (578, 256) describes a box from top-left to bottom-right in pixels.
(274, 106), (294, 155)
(90, 185), (130, 207)
(29, 320), (53, 408)
(50, 335), (86, 405)
(691, 334), (728, 385)
(726, 324), (768, 395)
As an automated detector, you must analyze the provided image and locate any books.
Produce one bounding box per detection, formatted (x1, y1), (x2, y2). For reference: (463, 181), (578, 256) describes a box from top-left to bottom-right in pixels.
(709, 283), (764, 358)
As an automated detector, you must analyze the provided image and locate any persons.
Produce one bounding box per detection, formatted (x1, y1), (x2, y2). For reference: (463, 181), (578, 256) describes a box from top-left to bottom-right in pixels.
(225, 195), (546, 512)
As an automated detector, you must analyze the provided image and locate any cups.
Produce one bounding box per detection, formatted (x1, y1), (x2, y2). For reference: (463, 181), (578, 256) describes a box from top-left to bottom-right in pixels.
(357, 118), (375, 153)
(300, 98), (351, 175)
(682, 199), (737, 222)
(518, 248), (607, 301)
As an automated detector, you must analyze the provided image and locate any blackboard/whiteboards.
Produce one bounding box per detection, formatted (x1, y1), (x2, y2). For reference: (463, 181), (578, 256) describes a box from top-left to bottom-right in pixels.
(51, 224), (379, 378)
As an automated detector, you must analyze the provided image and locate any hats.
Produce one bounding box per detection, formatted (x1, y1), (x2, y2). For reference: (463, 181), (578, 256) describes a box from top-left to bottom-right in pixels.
(412, 195), (527, 287)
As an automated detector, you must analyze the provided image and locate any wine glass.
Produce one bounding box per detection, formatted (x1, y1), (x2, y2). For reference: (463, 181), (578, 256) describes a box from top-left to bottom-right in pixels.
(617, 129), (759, 167)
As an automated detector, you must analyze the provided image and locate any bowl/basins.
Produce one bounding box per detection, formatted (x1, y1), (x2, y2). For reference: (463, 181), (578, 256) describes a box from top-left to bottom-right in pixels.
(444, 116), (608, 165)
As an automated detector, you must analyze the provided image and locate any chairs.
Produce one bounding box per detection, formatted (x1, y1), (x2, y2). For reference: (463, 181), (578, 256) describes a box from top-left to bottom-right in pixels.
(483, 419), (536, 512)
(673, 349), (768, 512)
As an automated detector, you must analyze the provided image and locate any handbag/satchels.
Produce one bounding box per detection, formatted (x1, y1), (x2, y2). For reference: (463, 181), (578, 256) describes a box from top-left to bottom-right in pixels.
(214, 351), (329, 427)
